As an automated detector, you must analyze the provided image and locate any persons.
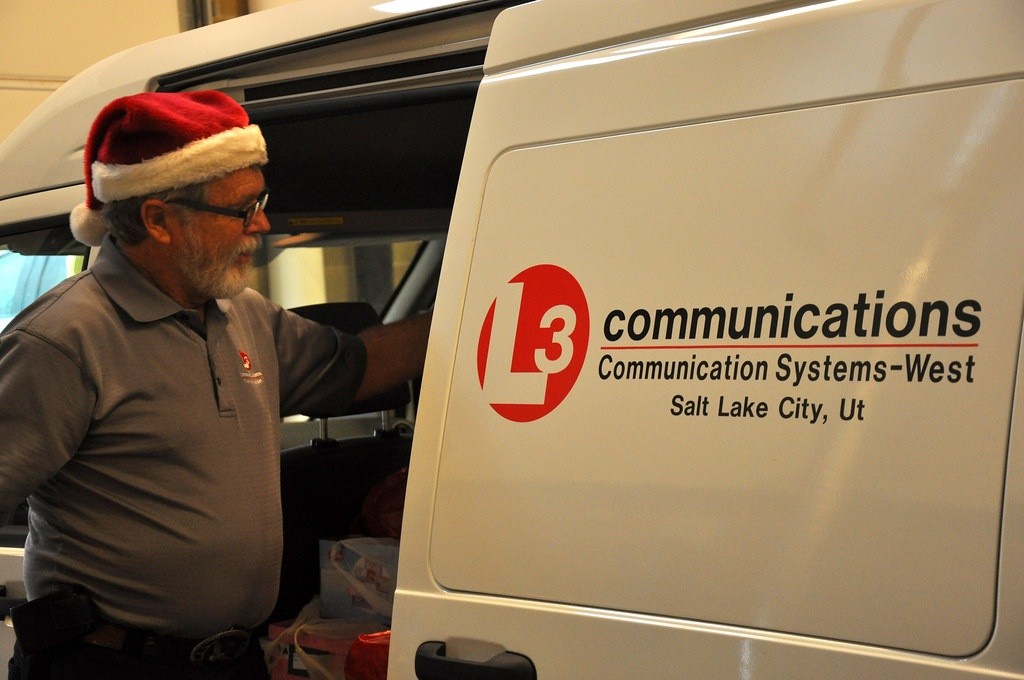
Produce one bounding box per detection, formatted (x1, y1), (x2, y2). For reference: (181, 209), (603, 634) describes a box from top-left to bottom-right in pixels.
(0, 88), (431, 680)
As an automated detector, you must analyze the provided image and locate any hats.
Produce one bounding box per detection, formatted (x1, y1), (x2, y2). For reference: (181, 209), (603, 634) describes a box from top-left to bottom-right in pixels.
(69, 90), (269, 247)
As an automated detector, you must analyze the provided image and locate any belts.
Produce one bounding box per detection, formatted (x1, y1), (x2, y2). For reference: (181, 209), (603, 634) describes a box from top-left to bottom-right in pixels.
(77, 620), (270, 671)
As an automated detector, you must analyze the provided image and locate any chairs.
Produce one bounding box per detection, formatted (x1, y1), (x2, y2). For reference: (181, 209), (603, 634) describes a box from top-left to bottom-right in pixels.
(279, 300), (415, 621)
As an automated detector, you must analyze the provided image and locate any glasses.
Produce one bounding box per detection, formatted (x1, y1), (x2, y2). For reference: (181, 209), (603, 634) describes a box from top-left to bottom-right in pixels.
(165, 193), (269, 227)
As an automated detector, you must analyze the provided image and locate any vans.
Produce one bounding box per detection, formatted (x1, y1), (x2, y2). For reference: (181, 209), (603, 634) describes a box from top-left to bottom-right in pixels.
(0, 0), (1024, 680)
